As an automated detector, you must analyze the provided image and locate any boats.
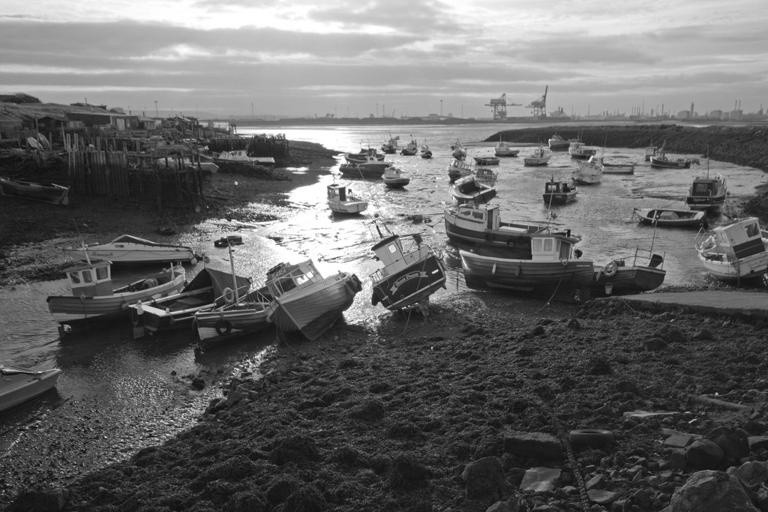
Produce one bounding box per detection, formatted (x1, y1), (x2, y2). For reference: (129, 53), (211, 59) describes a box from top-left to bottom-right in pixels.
(0, 176), (71, 207)
(47, 259), (188, 336)
(127, 267), (254, 339)
(217, 150), (275, 164)
(193, 260), (323, 353)
(265, 268), (362, 355)
(0, 365), (63, 413)
(63, 233), (195, 267)
(157, 156), (220, 174)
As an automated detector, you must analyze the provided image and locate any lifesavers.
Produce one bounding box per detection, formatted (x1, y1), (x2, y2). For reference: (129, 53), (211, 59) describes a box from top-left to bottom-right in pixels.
(604, 262), (617, 276)
(223, 287), (234, 303)
